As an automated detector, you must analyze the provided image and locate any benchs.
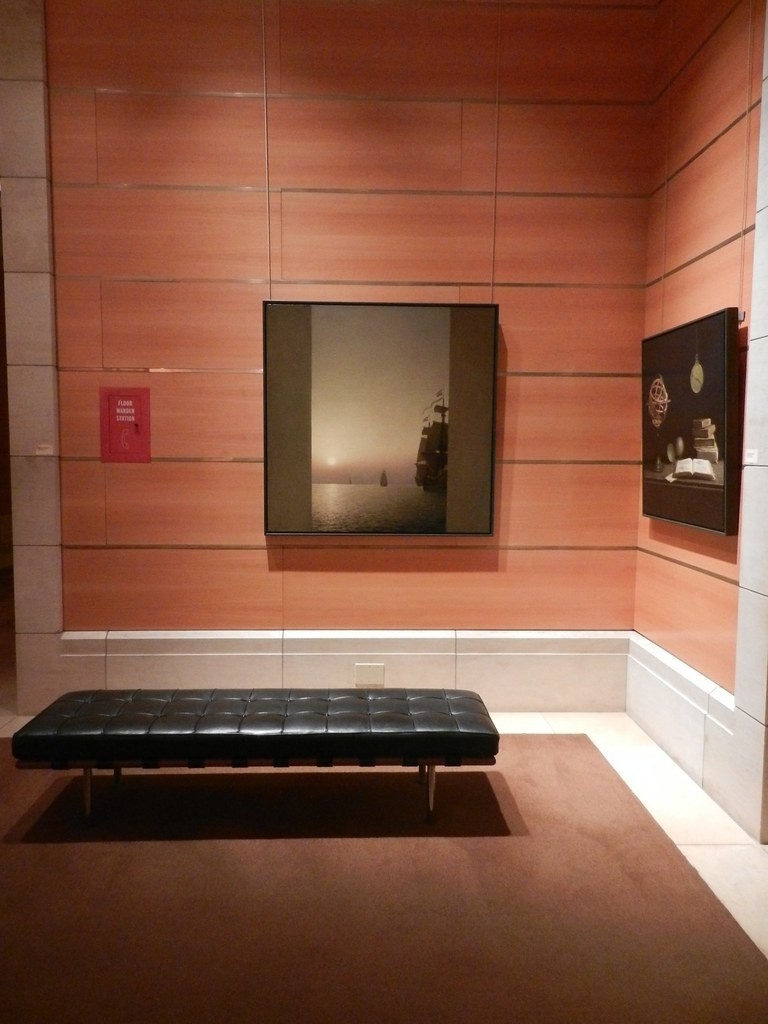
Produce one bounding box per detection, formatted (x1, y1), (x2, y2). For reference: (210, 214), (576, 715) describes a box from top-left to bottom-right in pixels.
(10, 687), (500, 822)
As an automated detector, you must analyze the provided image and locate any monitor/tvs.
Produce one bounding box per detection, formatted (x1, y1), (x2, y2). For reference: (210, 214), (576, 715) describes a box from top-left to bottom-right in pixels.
(641, 306), (741, 535)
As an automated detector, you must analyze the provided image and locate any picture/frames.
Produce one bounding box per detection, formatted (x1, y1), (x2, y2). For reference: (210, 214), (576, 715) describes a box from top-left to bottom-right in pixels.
(641, 307), (738, 536)
(261, 299), (498, 537)
